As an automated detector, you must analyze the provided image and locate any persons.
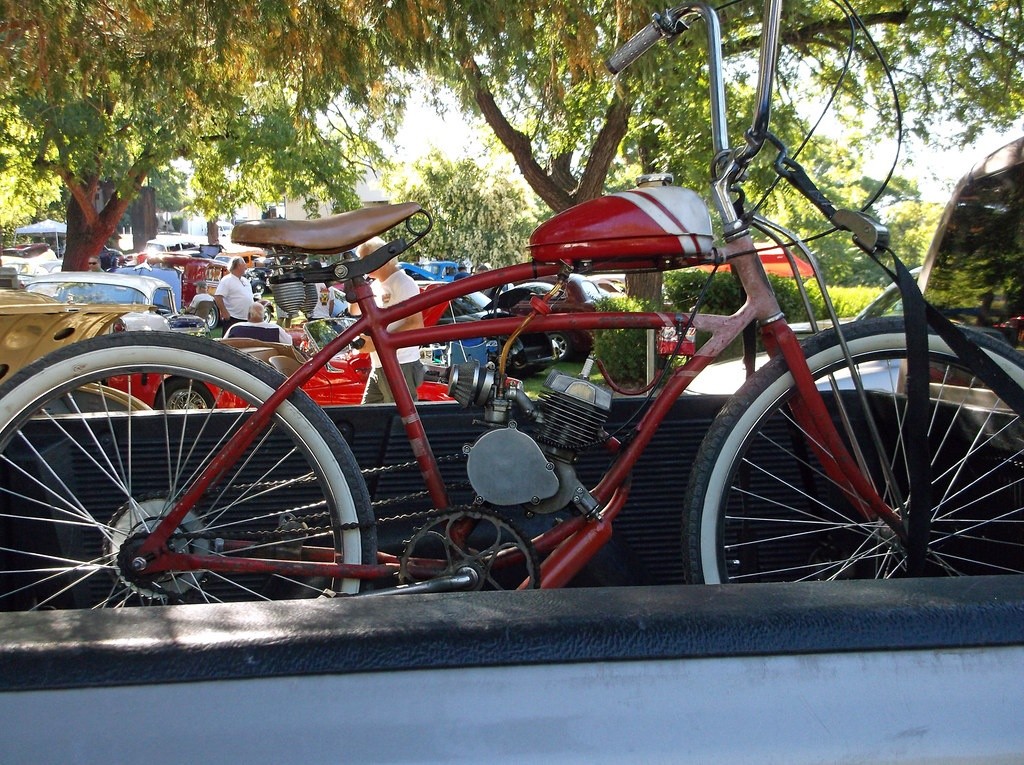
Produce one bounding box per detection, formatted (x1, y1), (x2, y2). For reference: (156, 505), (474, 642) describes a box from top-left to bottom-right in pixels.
(86, 256), (105, 273)
(308, 261), (349, 321)
(214, 256), (254, 338)
(276, 304), (298, 328)
(106, 256), (122, 273)
(348, 235), (424, 404)
(185, 281), (214, 315)
(454, 263), (470, 281)
(223, 301), (293, 345)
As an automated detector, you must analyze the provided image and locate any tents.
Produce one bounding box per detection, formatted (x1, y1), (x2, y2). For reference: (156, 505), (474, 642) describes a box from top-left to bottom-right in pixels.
(12, 219), (68, 260)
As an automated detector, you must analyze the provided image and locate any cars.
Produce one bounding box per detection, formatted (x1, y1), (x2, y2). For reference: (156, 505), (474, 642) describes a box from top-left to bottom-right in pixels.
(0, 236), (628, 413)
(683, 262), (923, 397)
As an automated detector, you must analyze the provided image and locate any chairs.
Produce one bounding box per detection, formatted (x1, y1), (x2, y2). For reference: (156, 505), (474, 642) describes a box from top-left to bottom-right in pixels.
(429, 340), (488, 366)
(228, 326), (280, 343)
(186, 301), (215, 338)
(269, 355), (301, 378)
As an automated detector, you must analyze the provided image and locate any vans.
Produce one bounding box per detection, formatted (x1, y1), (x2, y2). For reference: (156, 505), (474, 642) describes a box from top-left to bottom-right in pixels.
(896, 136), (1024, 411)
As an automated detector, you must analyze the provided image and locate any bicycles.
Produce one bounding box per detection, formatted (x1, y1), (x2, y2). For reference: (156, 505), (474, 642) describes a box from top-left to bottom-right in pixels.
(0, 2), (1022, 616)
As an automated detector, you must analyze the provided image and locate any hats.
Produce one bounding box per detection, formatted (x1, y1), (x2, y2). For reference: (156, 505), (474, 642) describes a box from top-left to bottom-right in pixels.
(458, 261), (472, 268)
(478, 263), (492, 269)
